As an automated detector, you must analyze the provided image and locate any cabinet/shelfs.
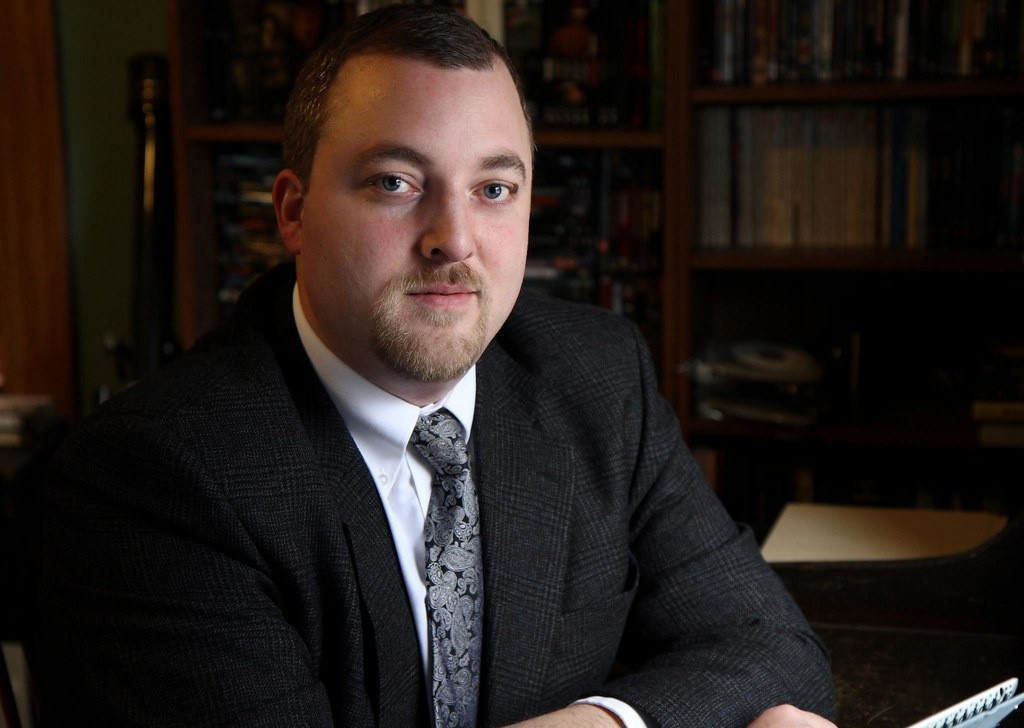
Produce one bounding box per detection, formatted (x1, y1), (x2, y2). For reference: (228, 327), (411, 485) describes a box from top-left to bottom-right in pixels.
(162, 0), (1024, 629)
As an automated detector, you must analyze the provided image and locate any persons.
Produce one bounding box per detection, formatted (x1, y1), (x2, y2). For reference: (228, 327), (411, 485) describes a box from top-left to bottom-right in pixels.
(20, 7), (836, 727)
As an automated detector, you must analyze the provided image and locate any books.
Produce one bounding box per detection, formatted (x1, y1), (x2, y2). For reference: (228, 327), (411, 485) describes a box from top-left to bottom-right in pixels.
(697, 1), (1008, 95)
(700, 103), (935, 253)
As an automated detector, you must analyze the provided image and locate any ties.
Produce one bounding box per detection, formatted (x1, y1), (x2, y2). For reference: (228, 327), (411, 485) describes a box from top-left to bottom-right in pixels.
(410, 410), (483, 728)
